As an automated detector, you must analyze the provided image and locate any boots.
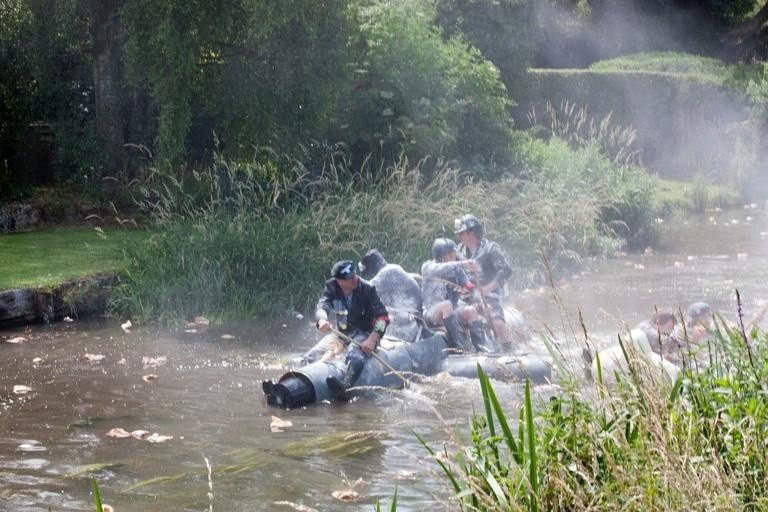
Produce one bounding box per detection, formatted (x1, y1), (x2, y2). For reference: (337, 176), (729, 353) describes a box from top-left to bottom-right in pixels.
(443, 315), (469, 350)
(327, 358), (364, 400)
(468, 322), (499, 353)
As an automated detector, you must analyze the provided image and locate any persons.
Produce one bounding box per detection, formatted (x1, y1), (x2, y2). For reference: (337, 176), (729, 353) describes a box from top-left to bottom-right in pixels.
(301, 215), (511, 406)
(627, 302), (732, 372)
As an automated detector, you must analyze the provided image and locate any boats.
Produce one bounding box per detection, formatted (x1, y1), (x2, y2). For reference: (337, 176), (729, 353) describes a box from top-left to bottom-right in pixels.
(262, 305), (551, 409)
(589, 341), (700, 393)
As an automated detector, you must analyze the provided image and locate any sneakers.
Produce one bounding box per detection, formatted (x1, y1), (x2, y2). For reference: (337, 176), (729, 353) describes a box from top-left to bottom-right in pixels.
(501, 342), (515, 353)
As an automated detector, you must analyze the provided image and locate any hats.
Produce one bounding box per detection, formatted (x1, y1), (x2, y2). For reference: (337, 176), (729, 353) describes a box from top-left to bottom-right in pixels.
(331, 260), (355, 278)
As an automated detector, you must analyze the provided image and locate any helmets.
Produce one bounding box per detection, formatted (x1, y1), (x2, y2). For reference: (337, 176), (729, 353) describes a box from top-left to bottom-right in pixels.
(686, 302), (710, 317)
(454, 215), (483, 235)
(431, 238), (458, 257)
